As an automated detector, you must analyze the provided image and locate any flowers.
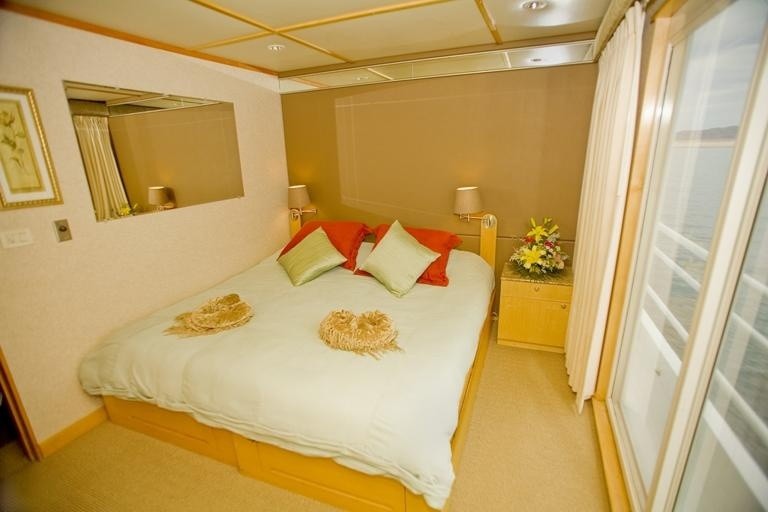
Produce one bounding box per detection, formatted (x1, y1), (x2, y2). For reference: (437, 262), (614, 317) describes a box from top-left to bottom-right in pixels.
(508, 217), (569, 282)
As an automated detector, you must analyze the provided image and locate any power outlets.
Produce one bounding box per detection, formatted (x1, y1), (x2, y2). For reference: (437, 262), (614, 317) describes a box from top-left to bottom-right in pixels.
(53, 219), (71, 241)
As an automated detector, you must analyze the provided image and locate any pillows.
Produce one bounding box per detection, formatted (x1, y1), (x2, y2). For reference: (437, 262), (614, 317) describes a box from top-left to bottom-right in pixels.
(277, 221), (463, 299)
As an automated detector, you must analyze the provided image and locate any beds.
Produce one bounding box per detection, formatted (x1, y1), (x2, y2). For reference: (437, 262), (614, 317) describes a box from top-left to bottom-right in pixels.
(79, 209), (497, 512)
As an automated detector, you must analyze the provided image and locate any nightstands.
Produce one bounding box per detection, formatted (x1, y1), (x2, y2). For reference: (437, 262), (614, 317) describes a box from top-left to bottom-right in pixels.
(496, 261), (572, 354)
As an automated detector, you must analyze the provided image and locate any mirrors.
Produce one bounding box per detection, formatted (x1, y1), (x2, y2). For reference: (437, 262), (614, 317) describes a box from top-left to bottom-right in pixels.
(62, 80), (245, 222)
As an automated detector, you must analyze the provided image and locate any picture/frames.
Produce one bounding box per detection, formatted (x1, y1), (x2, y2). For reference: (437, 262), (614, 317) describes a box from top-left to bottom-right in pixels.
(0, 85), (64, 210)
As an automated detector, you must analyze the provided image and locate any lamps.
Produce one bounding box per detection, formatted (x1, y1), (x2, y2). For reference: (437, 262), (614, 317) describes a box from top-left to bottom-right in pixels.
(452, 186), (483, 221)
(288, 185), (316, 221)
(148, 186), (174, 208)
